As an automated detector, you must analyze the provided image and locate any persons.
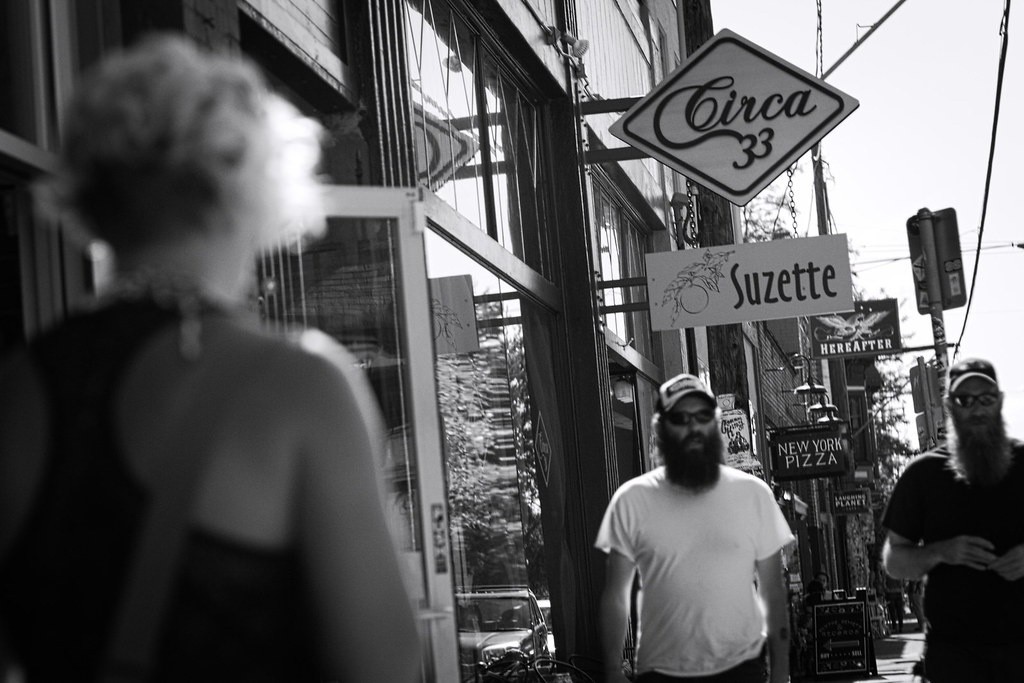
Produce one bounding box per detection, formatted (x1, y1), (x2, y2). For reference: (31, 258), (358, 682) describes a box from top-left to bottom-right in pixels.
(883, 361), (1024, 683)
(595, 374), (796, 683)
(885, 577), (926, 633)
(0, 41), (422, 682)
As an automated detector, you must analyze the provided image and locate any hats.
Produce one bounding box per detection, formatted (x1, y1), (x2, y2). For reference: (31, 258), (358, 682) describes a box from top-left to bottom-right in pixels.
(949, 357), (997, 391)
(657, 373), (717, 412)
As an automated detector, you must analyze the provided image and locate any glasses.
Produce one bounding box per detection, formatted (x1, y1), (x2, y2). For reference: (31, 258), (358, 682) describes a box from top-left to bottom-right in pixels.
(947, 392), (998, 407)
(665, 408), (716, 425)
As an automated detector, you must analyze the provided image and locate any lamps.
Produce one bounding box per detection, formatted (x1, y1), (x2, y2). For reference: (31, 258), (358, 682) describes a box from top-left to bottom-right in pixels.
(561, 34), (590, 56)
(793, 395), (843, 426)
(782, 376), (838, 411)
(776, 354), (828, 396)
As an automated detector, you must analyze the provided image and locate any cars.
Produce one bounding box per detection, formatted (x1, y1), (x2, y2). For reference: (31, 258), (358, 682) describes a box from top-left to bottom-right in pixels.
(534, 600), (558, 661)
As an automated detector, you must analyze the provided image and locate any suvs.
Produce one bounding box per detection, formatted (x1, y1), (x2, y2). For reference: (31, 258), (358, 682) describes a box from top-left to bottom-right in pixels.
(452, 584), (549, 679)
